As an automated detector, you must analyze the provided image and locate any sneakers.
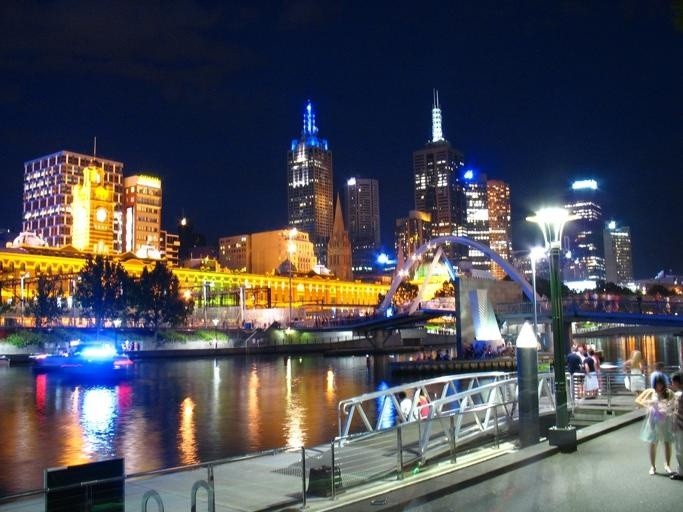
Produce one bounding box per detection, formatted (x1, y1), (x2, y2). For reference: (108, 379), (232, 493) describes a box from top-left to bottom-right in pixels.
(650, 466), (656, 475)
(664, 464), (682, 480)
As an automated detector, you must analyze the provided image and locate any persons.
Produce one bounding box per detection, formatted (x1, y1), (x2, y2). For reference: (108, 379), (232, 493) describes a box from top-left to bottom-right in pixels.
(635, 374), (675, 474)
(667, 372), (683, 479)
(650, 362), (671, 389)
(621, 350), (646, 409)
(541, 290), (682, 317)
(414, 337), (514, 361)
(312, 311), (369, 327)
(393, 391), (411, 425)
(567, 343), (601, 399)
(413, 388), (429, 417)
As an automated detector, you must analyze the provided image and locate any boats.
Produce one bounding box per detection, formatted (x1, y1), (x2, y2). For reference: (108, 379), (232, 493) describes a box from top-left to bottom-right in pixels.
(30, 337), (135, 379)
(384, 338), (556, 379)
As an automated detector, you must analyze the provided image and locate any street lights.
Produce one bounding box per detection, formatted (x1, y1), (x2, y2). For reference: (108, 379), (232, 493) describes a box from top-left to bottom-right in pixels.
(287, 228), (297, 329)
(201, 279), (215, 327)
(71, 275), (83, 327)
(514, 319), (543, 449)
(238, 278), (252, 332)
(526, 203), (585, 449)
(18, 271), (30, 329)
(528, 243), (548, 346)
